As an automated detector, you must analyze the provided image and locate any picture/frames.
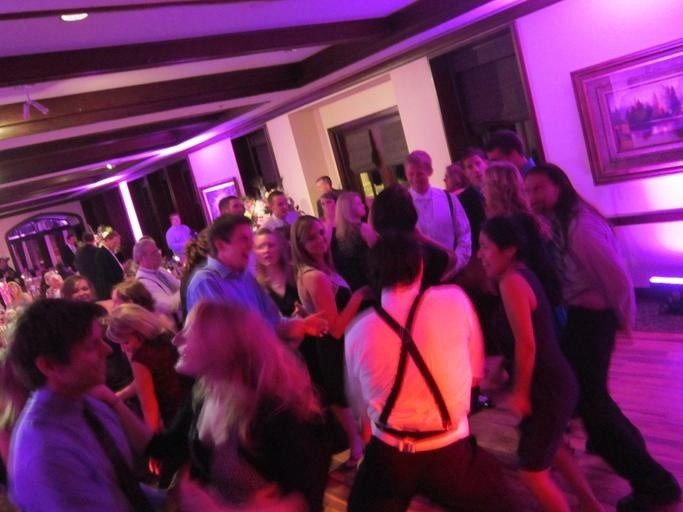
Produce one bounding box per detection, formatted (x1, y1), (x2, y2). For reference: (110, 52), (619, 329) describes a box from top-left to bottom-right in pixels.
(570, 37), (683, 187)
(200, 177), (241, 227)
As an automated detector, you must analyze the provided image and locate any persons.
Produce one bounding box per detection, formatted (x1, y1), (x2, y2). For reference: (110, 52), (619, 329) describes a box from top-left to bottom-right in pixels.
(0, 131), (682, 512)
